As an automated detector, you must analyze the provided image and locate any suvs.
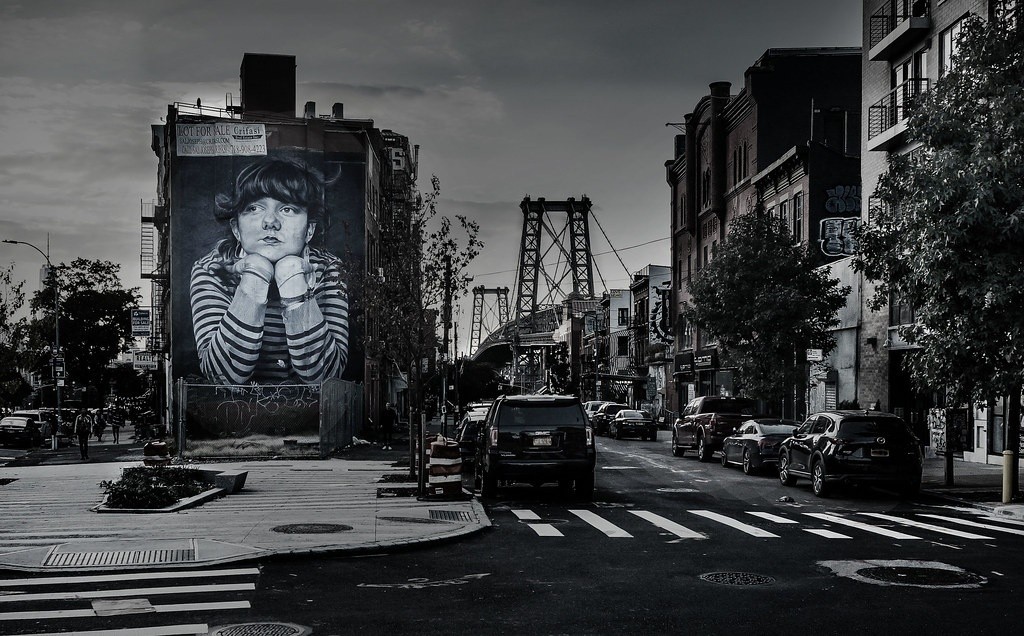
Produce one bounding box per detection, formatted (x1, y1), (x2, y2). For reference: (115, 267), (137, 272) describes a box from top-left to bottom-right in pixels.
(778, 411), (924, 497)
(468, 393), (597, 503)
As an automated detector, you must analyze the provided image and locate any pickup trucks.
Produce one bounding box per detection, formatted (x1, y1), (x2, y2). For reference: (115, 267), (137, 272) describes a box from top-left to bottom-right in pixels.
(672, 396), (767, 462)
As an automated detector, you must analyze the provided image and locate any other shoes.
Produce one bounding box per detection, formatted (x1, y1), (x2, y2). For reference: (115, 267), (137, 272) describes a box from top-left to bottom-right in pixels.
(382, 445), (386, 449)
(85, 454), (90, 459)
(113, 438), (116, 443)
(388, 446), (393, 450)
(81, 457), (84, 460)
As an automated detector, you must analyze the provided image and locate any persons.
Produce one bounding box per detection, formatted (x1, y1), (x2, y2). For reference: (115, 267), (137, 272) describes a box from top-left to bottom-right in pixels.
(378, 401), (399, 451)
(73, 403), (126, 460)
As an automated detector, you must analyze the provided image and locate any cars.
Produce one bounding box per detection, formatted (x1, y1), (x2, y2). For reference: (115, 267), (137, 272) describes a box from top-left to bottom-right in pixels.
(722, 418), (803, 475)
(581, 401), (658, 440)
(454, 400), (493, 475)
(0, 410), (50, 446)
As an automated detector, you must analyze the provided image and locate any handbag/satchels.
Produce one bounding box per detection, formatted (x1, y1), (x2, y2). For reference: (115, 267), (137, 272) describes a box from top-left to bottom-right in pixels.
(99, 419), (106, 429)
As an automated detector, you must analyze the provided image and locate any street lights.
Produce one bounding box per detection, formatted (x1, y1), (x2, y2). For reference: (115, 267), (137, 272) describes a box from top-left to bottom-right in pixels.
(1, 239), (61, 417)
